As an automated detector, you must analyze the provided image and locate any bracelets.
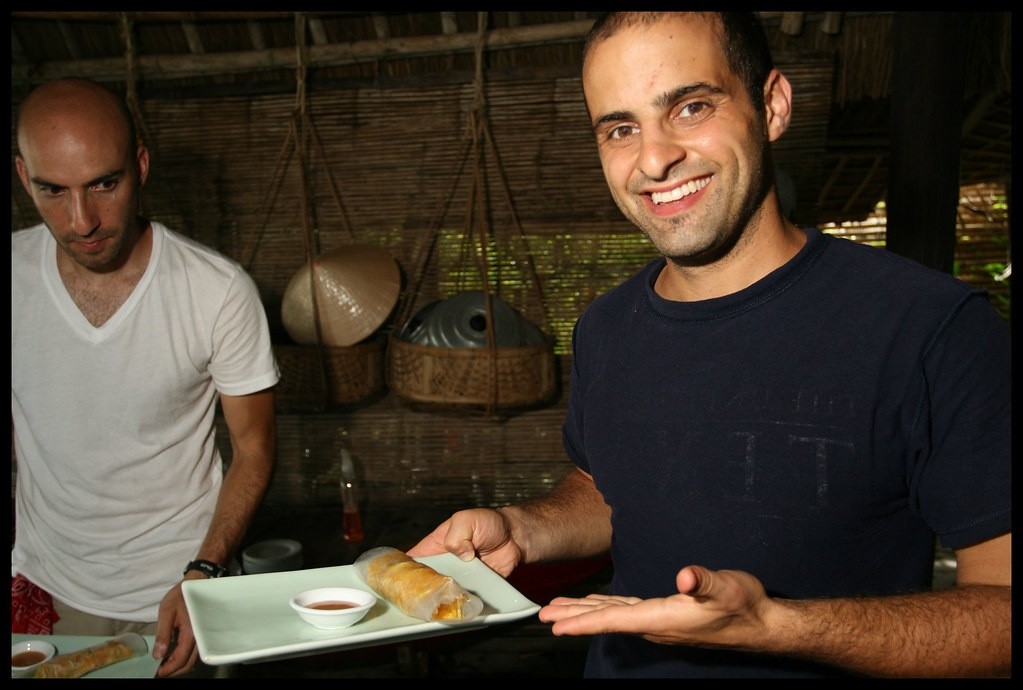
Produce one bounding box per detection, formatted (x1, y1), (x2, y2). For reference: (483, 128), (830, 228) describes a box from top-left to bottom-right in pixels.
(184, 559), (229, 580)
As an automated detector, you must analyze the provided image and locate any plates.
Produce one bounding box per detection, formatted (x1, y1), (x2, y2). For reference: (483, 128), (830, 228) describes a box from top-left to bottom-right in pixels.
(11, 633), (164, 679)
(181, 552), (542, 665)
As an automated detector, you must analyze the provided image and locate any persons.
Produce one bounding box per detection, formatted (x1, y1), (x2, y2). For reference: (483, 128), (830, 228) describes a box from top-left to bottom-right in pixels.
(405, 12), (1011, 678)
(12, 78), (281, 679)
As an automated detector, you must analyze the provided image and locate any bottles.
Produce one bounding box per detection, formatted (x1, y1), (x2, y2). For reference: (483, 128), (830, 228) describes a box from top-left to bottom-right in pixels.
(340, 449), (364, 542)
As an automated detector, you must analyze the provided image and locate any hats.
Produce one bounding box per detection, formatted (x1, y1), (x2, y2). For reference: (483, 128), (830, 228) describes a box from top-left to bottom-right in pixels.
(282, 241), (402, 346)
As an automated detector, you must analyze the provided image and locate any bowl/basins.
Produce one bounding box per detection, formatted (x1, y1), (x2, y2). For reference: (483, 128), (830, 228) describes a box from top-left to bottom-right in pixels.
(11, 641), (55, 677)
(291, 588), (377, 631)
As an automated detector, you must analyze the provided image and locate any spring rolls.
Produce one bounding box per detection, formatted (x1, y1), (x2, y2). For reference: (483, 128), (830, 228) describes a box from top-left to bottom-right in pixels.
(354, 546), (484, 624)
(36, 633), (149, 677)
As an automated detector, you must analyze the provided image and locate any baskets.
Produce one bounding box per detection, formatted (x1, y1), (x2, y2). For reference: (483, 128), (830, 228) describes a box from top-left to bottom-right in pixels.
(269, 338), (383, 409)
(388, 337), (558, 412)
